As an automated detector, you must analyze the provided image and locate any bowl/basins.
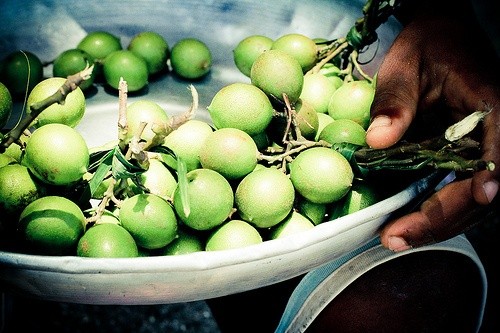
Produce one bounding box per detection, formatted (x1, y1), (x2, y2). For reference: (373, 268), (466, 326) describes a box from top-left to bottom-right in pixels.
(1, 0), (456, 306)
